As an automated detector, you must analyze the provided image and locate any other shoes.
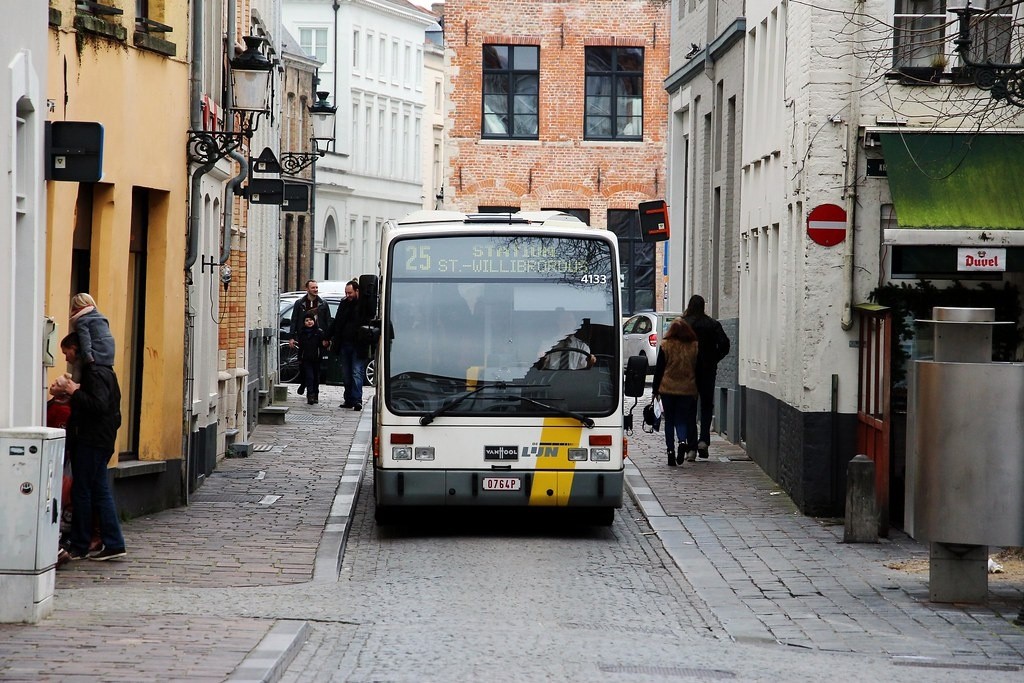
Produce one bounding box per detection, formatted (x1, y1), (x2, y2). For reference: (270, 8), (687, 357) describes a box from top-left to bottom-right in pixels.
(339, 400), (354, 408)
(698, 441), (709, 459)
(667, 450), (677, 466)
(686, 450), (698, 461)
(88, 537), (103, 551)
(54, 395), (71, 406)
(354, 403), (362, 411)
(677, 442), (687, 464)
(297, 381), (307, 395)
(306, 390), (319, 405)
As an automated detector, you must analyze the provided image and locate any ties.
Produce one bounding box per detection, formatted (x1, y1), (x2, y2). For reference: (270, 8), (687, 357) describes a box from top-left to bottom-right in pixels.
(560, 338), (572, 369)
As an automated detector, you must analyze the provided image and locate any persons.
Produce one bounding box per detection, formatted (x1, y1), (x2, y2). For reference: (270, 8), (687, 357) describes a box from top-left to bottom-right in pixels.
(289, 279), (331, 404)
(681, 295), (731, 462)
(67, 292), (115, 432)
(46, 372), (71, 428)
(329, 281), (370, 409)
(536, 310), (597, 369)
(652, 317), (699, 466)
(60, 333), (127, 561)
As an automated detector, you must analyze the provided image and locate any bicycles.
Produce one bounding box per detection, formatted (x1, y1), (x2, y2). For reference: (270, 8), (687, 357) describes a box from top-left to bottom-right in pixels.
(277, 339), (301, 382)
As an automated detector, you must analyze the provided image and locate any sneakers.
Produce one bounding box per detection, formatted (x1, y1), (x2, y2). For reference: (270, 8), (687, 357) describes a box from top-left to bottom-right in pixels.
(58, 539), (90, 560)
(89, 545), (127, 561)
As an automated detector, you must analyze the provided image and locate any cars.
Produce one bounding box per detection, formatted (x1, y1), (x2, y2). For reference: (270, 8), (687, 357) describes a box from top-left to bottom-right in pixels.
(279, 294), (377, 385)
(623, 311), (658, 373)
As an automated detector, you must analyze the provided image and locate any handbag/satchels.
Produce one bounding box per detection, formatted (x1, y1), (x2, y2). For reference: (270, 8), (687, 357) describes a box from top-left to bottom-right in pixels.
(641, 396), (664, 433)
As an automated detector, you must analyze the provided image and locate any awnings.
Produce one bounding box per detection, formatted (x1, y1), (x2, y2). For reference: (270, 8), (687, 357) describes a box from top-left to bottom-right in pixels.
(884, 133), (1024, 245)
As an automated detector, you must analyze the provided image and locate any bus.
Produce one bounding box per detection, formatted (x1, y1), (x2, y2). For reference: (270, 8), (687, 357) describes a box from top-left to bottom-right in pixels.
(359, 207), (648, 529)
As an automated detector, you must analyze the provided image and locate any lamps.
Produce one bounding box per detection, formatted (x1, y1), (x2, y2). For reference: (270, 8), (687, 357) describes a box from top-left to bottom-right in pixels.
(280, 92), (338, 174)
(187, 33), (275, 174)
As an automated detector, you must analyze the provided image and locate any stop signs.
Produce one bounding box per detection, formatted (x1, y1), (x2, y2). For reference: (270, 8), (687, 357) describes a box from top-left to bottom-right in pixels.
(807, 203), (847, 247)
